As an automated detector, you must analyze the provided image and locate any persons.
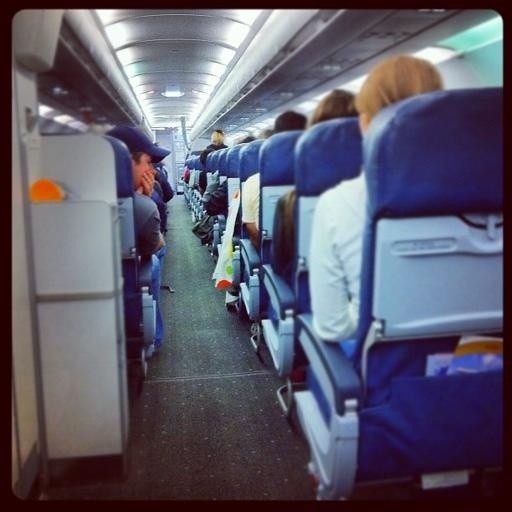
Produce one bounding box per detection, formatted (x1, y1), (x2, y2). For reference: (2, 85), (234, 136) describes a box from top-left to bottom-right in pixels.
(138, 110), (309, 322)
(104, 124), (170, 363)
(307, 54), (446, 350)
(267, 84), (361, 278)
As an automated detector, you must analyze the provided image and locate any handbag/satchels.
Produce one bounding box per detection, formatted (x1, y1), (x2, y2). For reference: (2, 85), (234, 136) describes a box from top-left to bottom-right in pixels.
(192, 215), (215, 243)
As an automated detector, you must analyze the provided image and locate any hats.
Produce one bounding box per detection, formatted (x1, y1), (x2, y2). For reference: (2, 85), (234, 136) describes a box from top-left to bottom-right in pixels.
(104, 125), (171, 163)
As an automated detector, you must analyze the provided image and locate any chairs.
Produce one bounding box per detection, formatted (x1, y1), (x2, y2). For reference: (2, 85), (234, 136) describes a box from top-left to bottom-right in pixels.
(105, 133), (158, 381)
(185, 85), (503, 501)
